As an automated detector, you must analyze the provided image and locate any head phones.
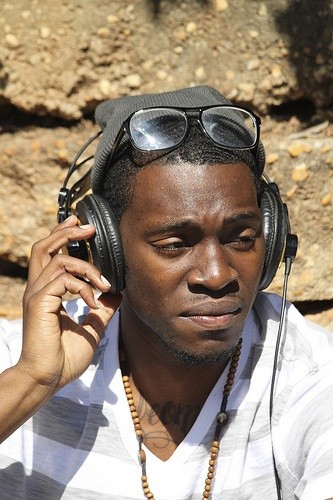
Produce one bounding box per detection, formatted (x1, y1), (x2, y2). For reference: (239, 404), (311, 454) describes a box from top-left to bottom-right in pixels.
(57, 130), (291, 295)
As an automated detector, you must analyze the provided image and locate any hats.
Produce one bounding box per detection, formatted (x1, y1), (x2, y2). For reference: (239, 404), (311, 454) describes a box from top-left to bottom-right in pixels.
(90, 87), (266, 195)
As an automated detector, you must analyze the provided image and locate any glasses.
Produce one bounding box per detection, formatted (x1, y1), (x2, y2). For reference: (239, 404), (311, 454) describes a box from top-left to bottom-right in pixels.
(100, 105), (261, 181)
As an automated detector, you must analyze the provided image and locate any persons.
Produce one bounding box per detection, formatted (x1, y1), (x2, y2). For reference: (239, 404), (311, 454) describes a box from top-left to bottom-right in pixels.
(0, 86), (333, 500)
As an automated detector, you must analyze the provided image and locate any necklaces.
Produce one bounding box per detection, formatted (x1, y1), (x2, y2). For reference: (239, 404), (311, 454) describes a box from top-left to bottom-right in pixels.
(119, 336), (245, 500)
